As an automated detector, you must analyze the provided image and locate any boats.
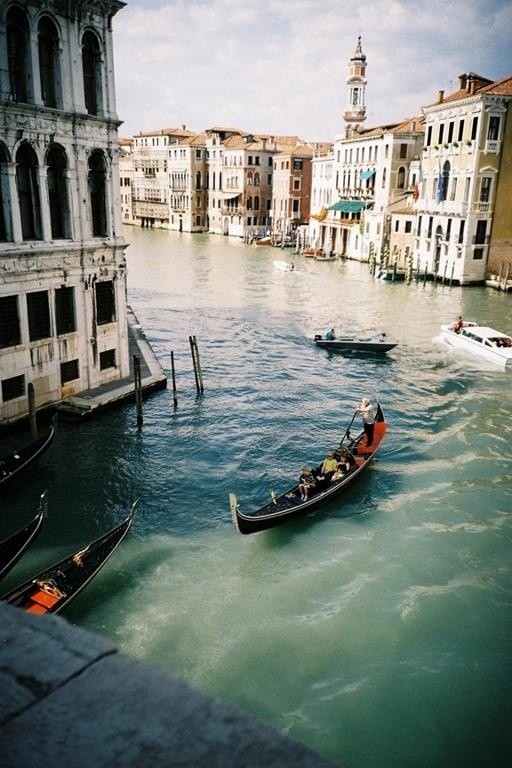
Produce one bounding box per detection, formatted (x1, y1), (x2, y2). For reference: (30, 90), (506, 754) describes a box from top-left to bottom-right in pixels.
(314, 334), (397, 355)
(439, 320), (512, 366)
(1, 427), (139, 616)
(274, 259), (295, 271)
(229, 406), (386, 535)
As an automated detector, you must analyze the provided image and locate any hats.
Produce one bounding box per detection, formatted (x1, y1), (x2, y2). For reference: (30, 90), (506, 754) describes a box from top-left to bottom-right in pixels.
(327, 452), (335, 457)
(301, 466), (312, 472)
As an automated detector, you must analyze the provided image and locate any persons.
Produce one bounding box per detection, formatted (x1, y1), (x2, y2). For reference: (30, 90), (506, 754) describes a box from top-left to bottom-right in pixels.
(454, 315), (464, 334)
(324, 328), (337, 340)
(316, 448), (338, 482)
(330, 455), (350, 483)
(297, 466), (318, 502)
(351, 397), (376, 449)
